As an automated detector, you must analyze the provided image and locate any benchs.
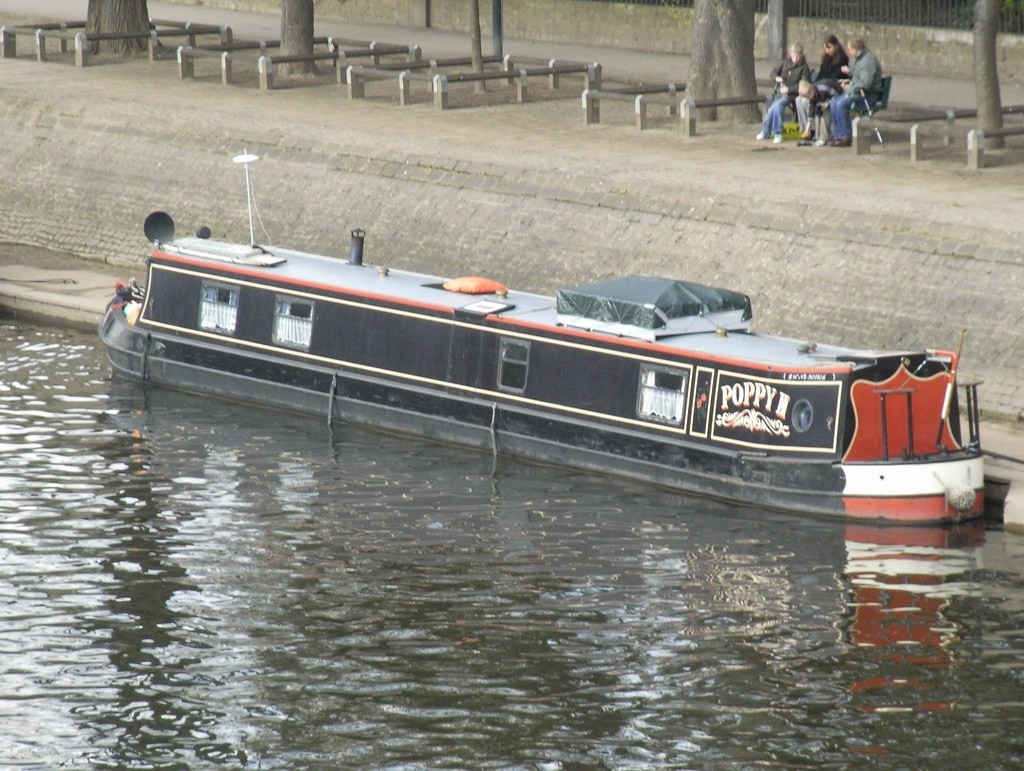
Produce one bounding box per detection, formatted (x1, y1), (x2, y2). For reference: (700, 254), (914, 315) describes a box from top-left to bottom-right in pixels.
(772, 74), (891, 123)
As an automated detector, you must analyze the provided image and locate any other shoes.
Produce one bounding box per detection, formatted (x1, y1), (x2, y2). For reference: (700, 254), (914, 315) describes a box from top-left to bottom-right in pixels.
(796, 134), (813, 146)
(756, 131), (770, 139)
(773, 132), (782, 143)
(814, 138), (827, 146)
(831, 134), (852, 146)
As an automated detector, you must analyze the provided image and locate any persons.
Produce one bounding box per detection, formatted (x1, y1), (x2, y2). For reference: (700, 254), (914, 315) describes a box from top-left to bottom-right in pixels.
(756, 34), (883, 147)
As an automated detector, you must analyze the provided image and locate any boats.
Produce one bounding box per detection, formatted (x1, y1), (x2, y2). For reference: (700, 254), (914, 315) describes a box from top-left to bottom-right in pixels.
(95, 146), (987, 533)
